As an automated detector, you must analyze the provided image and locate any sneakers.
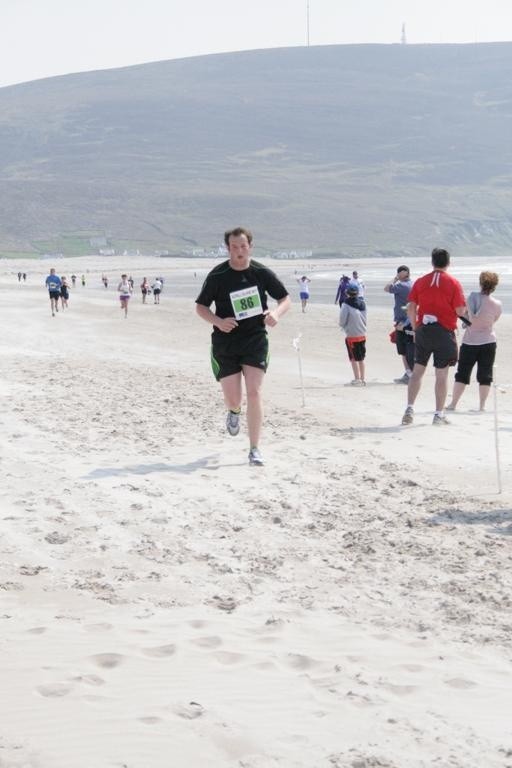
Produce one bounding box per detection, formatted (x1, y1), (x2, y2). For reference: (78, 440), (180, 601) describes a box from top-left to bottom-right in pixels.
(402, 408), (413, 424)
(432, 413), (451, 425)
(393, 374), (409, 384)
(344, 379), (362, 387)
(226, 407), (240, 436)
(249, 447), (264, 467)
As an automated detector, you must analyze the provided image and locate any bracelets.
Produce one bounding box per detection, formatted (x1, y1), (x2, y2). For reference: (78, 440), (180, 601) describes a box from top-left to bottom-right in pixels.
(395, 276), (400, 280)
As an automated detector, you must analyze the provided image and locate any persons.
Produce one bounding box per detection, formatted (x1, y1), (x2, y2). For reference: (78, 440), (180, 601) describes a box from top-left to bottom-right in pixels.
(444, 270), (503, 412)
(196, 228), (294, 466)
(338, 285), (367, 388)
(351, 271), (365, 301)
(297, 275), (312, 314)
(335, 274), (351, 309)
(401, 248), (466, 427)
(194, 271), (196, 280)
(383, 265), (415, 386)
(18, 267), (164, 318)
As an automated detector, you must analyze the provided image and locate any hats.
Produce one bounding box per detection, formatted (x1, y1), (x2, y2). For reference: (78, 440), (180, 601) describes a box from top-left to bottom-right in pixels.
(348, 283), (358, 293)
